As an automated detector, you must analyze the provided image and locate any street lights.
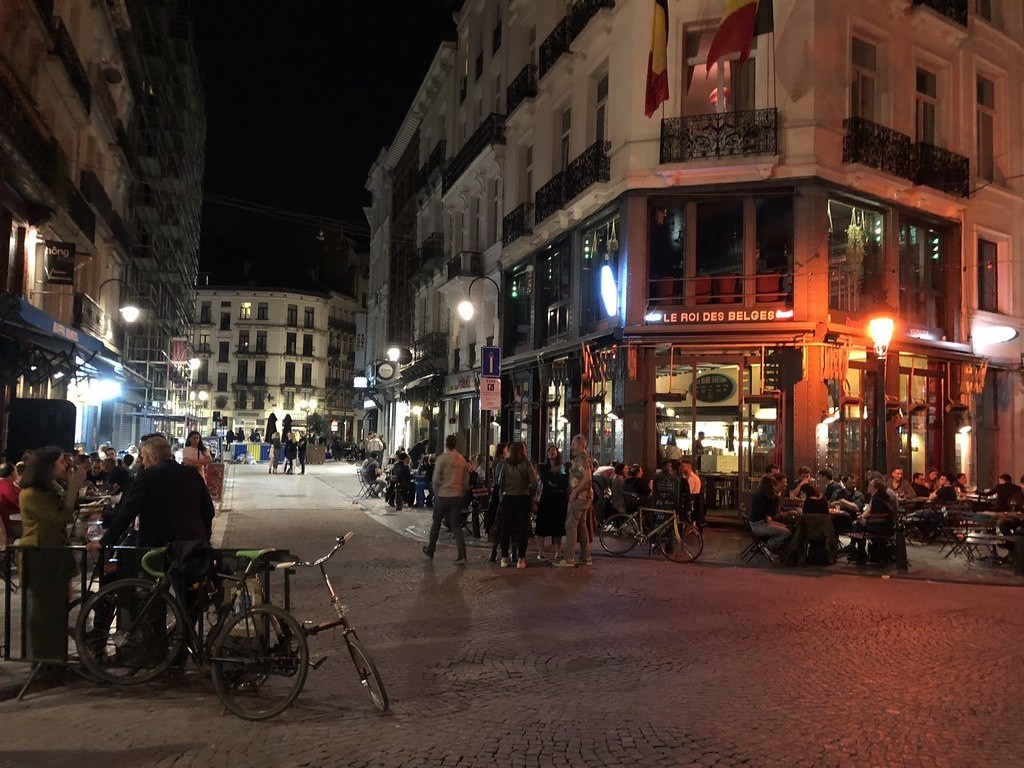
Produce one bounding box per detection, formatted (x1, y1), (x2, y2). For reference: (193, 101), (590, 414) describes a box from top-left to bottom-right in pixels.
(300, 399), (317, 437)
(860, 312), (898, 474)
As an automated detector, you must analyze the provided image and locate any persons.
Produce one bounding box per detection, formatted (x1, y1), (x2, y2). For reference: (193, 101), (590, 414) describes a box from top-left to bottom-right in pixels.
(238, 427), (245, 442)
(748, 464), (1024, 575)
(295, 431), (307, 475)
(593, 459), (704, 537)
(551, 435), (594, 567)
(0, 430), (215, 683)
(423, 435), (469, 565)
(364, 432), (386, 468)
(252, 430), (260, 442)
(269, 432), (281, 474)
(696, 431), (705, 450)
(486, 442), (569, 569)
(282, 432), (297, 474)
(360, 439), (494, 528)
(226, 430), (234, 450)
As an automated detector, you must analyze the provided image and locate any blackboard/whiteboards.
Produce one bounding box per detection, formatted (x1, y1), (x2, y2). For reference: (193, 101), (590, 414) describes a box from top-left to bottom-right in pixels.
(649, 476), (679, 544)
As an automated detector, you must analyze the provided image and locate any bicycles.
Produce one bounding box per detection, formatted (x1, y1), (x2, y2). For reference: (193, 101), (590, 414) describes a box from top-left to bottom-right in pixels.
(599, 492), (705, 564)
(74, 530), (389, 721)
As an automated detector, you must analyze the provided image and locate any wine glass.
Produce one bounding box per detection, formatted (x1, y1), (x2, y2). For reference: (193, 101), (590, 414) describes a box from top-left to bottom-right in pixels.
(96, 480), (103, 495)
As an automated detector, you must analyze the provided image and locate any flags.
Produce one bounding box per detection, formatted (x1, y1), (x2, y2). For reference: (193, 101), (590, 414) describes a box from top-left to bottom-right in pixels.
(645, 0), (669, 118)
(706, 0), (760, 76)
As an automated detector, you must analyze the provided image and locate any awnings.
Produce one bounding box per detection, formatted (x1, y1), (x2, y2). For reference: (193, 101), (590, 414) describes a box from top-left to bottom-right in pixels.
(0, 290), (153, 388)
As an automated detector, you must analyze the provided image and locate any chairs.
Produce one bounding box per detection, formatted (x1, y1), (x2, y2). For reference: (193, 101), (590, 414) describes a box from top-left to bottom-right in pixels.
(736, 487), (1024, 570)
(653, 271), (781, 305)
(356, 469), (378, 499)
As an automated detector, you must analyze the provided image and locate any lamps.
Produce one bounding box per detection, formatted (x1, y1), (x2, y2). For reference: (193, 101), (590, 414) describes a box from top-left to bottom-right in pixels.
(821, 410), (836, 423)
(99, 278), (138, 322)
(839, 397), (968, 413)
(504, 390), (606, 410)
(606, 406), (622, 420)
(458, 276), (500, 323)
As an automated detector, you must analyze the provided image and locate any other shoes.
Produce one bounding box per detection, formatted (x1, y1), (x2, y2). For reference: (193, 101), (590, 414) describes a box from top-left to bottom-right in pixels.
(501, 557), (508, 567)
(764, 546), (780, 561)
(555, 554), (563, 558)
(489, 553), (495, 561)
(422, 545), (433, 560)
(538, 555), (544, 559)
(269, 469), (304, 475)
(510, 552), (517, 562)
(453, 558), (467, 565)
(517, 559), (527, 568)
(839, 542), (881, 565)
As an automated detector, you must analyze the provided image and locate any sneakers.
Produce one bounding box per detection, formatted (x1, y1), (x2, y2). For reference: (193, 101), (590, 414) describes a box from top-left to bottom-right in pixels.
(552, 559), (575, 566)
(574, 558), (592, 565)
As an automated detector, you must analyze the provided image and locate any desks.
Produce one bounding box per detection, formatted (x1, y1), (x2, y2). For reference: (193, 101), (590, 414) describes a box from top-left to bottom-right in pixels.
(449, 508), (488, 539)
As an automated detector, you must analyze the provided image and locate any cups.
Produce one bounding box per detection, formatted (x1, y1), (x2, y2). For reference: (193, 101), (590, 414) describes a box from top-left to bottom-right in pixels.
(87, 524), (103, 542)
(789, 491), (794, 500)
(835, 505), (840, 512)
(899, 490), (904, 500)
(79, 486), (87, 496)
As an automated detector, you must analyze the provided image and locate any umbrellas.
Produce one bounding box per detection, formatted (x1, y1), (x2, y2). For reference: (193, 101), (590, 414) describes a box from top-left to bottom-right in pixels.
(281, 415), (292, 443)
(265, 413), (277, 444)
